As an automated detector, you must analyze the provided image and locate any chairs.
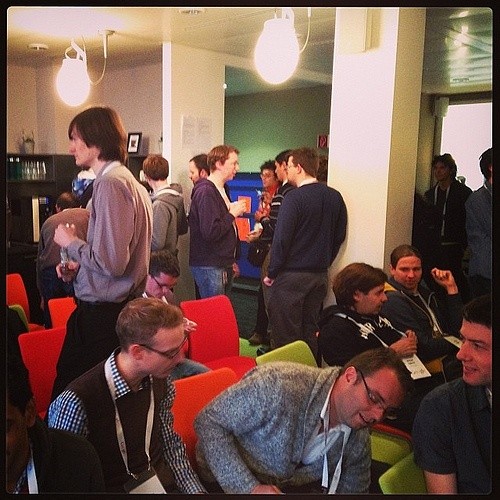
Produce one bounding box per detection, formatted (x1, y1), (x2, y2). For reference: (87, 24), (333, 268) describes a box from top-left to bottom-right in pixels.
(7, 272), (427, 494)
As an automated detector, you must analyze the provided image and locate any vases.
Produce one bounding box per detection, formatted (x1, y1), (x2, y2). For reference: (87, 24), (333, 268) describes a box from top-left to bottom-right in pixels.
(19, 142), (34, 155)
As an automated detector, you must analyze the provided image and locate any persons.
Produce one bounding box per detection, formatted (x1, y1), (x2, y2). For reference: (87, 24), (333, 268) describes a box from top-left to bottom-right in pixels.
(380, 244), (461, 386)
(36, 193), (90, 330)
(13, 298), (208, 494)
(319, 263), (448, 437)
(193, 349), (412, 494)
(143, 154), (188, 293)
(44, 107), (153, 426)
(413, 293), (492, 494)
(189, 153), (210, 184)
(188, 146), (247, 299)
(263, 147), (347, 368)
(424, 153), (473, 289)
(245, 149), (297, 344)
(142, 249), (180, 304)
(466, 148), (492, 300)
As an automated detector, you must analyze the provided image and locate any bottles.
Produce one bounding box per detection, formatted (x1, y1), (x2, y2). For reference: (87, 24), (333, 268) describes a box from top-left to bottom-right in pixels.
(59, 226), (77, 283)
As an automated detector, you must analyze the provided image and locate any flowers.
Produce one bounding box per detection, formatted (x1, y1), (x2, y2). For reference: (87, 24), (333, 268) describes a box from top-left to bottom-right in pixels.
(14, 128), (34, 143)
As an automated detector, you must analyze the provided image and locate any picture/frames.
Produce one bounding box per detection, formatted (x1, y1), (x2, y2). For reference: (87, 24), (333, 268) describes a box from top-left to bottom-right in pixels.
(127, 132), (142, 154)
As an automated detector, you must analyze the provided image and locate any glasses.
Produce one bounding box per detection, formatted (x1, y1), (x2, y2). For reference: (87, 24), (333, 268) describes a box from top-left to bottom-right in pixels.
(139, 330), (188, 361)
(148, 272), (177, 291)
(356, 369), (400, 420)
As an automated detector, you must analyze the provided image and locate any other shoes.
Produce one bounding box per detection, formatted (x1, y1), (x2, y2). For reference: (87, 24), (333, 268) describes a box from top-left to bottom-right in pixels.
(257, 344), (272, 355)
(249, 333), (263, 344)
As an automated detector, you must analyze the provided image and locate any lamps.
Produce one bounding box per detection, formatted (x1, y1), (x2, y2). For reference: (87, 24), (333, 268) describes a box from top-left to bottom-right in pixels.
(55, 30), (115, 105)
(254, 7), (312, 83)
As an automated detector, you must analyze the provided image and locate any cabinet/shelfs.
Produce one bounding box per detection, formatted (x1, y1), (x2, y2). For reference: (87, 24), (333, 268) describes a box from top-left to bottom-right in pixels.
(8, 152), (82, 255)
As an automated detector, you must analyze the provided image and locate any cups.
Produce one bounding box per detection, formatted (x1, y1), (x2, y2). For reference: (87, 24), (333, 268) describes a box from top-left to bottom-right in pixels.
(234, 201), (244, 216)
(21, 161), (46, 180)
